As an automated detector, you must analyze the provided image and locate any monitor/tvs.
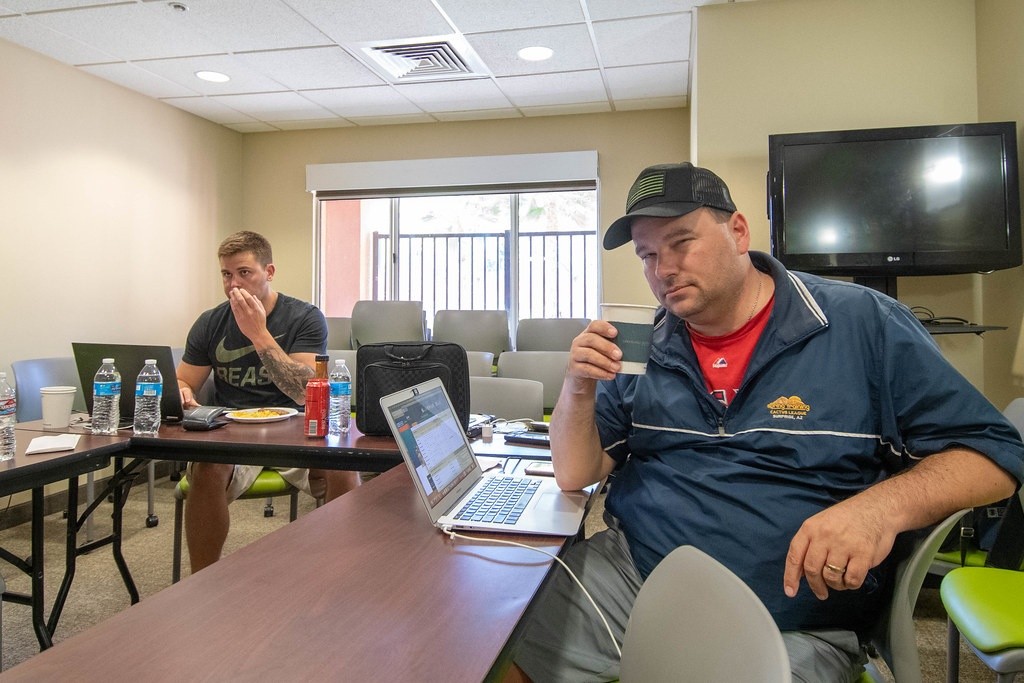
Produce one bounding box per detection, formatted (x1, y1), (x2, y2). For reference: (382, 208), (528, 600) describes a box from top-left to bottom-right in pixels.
(768, 121), (1024, 277)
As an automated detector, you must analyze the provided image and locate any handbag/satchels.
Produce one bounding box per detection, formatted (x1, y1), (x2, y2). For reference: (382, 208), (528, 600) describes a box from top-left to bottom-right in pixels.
(354, 339), (472, 435)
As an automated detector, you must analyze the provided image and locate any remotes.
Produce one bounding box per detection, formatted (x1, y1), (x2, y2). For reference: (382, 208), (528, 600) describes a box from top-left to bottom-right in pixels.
(504, 430), (551, 446)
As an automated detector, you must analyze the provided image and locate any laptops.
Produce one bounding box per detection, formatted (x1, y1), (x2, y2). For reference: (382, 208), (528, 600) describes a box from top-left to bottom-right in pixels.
(72, 342), (236, 425)
(379, 377), (593, 537)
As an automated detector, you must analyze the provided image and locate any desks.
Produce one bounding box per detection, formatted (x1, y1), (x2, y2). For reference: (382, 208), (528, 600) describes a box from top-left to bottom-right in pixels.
(0, 408), (610, 683)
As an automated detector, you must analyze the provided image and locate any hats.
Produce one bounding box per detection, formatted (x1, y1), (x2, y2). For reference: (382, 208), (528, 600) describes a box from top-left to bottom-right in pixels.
(603, 162), (738, 251)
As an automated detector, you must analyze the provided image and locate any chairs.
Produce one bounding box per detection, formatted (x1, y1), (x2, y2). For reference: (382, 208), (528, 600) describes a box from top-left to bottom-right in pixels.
(614, 393), (1024, 683)
(11, 300), (593, 585)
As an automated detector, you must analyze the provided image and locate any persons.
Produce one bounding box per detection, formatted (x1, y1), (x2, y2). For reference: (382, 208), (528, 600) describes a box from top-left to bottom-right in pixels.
(175, 231), (361, 576)
(512, 162), (1023, 683)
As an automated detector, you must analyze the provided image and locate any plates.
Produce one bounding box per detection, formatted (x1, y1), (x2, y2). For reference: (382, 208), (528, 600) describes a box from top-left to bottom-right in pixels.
(225, 407), (299, 422)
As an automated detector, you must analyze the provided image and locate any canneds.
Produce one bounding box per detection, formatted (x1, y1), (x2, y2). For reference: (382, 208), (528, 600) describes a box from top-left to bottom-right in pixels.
(303, 379), (331, 438)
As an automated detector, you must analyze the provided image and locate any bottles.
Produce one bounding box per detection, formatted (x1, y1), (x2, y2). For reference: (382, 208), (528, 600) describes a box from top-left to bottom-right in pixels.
(0, 372), (18, 462)
(92, 358), (122, 435)
(314, 356), (330, 378)
(133, 358), (163, 435)
(328, 359), (353, 434)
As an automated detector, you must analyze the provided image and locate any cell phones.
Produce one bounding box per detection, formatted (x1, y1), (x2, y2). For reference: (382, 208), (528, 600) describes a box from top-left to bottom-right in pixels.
(524, 461), (555, 476)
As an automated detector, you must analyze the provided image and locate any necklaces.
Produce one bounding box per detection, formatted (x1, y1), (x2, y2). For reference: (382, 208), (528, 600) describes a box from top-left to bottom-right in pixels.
(749, 268), (762, 321)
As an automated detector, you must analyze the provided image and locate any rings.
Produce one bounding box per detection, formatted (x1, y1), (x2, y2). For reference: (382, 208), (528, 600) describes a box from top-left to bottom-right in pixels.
(825, 562), (846, 574)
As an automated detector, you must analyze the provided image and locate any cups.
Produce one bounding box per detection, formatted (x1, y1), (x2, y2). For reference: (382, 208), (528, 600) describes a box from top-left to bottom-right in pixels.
(601, 302), (658, 375)
(39, 386), (77, 430)
